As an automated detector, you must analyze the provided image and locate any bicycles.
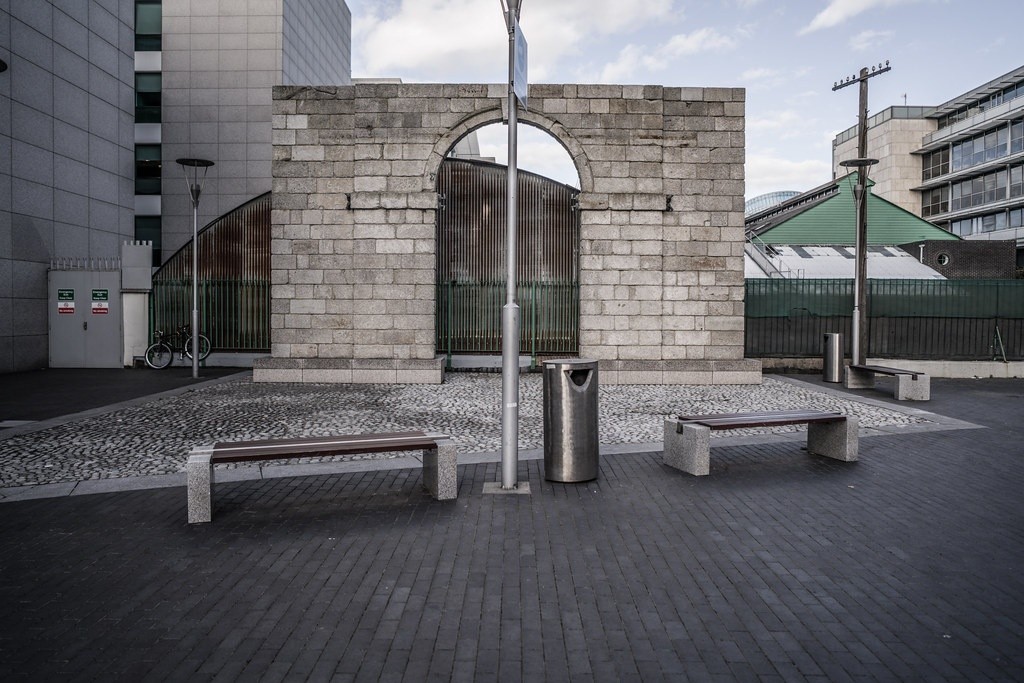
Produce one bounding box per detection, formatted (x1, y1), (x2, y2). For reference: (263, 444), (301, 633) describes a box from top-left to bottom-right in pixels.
(144, 324), (212, 370)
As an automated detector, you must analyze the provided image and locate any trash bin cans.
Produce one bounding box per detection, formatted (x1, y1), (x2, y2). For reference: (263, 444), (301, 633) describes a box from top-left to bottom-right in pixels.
(822, 333), (844, 383)
(542, 358), (600, 483)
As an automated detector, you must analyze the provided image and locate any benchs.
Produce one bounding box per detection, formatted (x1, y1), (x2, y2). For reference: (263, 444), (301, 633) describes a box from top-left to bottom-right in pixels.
(189, 430), (457, 524)
(662, 411), (861, 477)
(844, 364), (931, 400)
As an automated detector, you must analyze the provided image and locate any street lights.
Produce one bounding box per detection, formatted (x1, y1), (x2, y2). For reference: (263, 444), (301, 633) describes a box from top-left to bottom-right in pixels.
(176, 158), (216, 379)
(839, 157), (880, 366)
